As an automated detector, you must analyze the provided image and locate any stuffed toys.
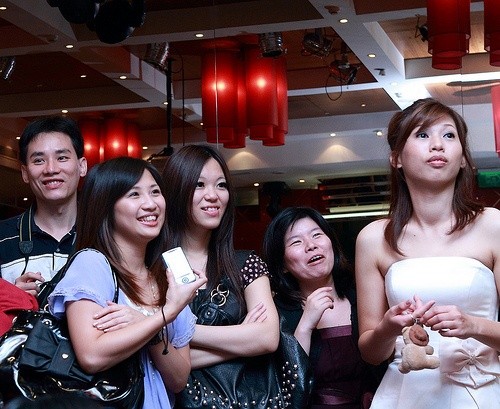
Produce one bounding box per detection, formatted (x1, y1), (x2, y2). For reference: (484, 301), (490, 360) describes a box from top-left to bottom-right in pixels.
(398, 325), (440, 374)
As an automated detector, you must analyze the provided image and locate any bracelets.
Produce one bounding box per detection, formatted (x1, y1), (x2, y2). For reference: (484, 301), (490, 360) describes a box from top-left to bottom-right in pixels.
(161, 307), (169, 356)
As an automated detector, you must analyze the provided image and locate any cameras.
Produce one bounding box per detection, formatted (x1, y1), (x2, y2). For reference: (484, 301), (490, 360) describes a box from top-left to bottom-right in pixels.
(161, 246), (198, 286)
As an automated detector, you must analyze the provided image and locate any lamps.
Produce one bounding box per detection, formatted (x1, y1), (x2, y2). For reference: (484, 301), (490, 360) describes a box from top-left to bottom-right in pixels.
(142, 41), (184, 76)
(201, 47), (288, 149)
(147, 57), (175, 171)
(77, 114), (144, 192)
(47, 0), (148, 44)
(413, 14), (429, 43)
(324, 40), (364, 102)
(299, 26), (338, 61)
(262, 182), (291, 218)
(256, 33), (287, 60)
(427, 0), (500, 70)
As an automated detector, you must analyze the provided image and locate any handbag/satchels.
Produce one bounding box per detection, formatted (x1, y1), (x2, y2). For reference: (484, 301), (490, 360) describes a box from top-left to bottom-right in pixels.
(1, 248), (145, 408)
(177, 251), (315, 409)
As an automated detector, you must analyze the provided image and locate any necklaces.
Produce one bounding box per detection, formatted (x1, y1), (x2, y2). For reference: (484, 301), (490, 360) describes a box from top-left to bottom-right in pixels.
(136, 271), (159, 317)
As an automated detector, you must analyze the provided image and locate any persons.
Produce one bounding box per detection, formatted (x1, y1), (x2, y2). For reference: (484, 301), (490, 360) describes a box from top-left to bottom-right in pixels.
(147, 145), (279, 409)
(0, 117), (87, 294)
(355, 98), (500, 409)
(47, 157), (208, 409)
(265, 206), (389, 409)
